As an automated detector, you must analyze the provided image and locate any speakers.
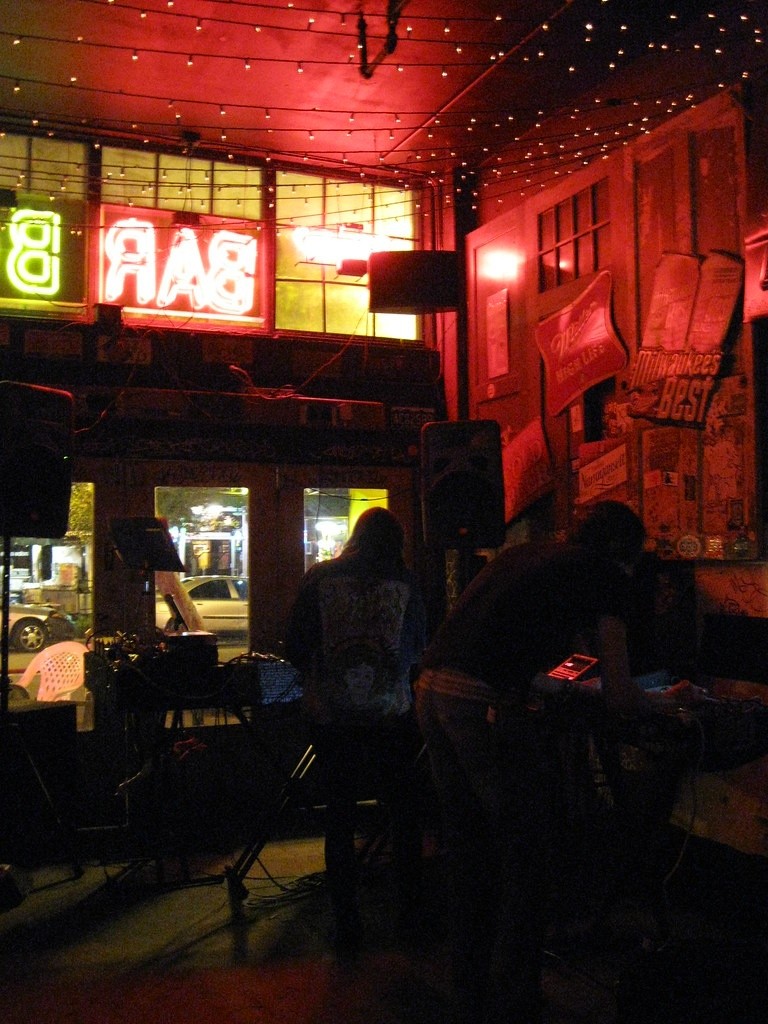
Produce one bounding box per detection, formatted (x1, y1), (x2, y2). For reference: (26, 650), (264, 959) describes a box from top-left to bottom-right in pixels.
(0, 381), (77, 538)
(369, 250), (464, 314)
(422, 421), (506, 550)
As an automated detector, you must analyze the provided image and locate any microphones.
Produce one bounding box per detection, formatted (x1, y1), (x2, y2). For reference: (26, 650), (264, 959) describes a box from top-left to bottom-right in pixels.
(165, 594), (188, 630)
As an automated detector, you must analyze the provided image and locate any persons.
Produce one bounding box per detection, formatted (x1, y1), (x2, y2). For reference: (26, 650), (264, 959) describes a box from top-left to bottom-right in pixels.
(286, 503), (705, 1024)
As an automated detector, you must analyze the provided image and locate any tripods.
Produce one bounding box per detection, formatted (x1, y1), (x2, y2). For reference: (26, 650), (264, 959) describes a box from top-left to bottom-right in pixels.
(0, 536), (85, 877)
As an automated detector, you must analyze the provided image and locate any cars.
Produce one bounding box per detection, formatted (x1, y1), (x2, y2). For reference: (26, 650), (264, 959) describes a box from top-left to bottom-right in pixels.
(0, 597), (59, 653)
(155, 574), (249, 638)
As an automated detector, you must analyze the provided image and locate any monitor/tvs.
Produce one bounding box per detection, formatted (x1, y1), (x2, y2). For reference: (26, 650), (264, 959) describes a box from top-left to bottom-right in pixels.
(700, 613), (768, 686)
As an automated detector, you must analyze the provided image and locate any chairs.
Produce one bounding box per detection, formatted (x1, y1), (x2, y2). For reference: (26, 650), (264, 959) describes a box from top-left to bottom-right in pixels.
(15, 641), (89, 702)
